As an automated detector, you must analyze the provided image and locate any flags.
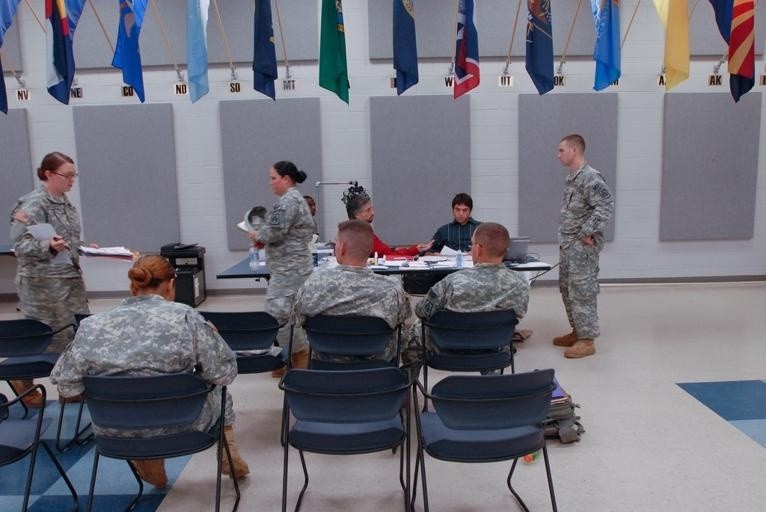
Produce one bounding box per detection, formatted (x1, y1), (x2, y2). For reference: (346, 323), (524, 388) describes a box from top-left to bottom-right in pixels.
(653, 0), (693, 92)
(251, 0), (278, 103)
(592, 0), (621, 91)
(111, 0), (149, 104)
(524, 0), (555, 95)
(319, 0), (351, 107)
(44, 0), (86, 108)
(392, 0), (419, 95)
(453, 0), (480, 99)
(0, 0), (21, 115)
(708, 0), (757, 103)
(185, 0), (211, 104)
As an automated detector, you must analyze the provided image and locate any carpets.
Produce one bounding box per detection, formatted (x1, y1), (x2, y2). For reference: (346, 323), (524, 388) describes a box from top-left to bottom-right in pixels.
(677, 380), (766, 449)
(1, 398), (191, 512)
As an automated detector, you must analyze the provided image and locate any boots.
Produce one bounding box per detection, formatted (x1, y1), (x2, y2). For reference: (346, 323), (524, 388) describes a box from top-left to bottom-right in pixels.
(133, 457), (167, 488)
(293, 344), (314, 370)
(220, 426), (249, 478)
(272, 368), (284, 378)
(564, 337), (595, 358)
(11, 379), (43, 408)
(58, 393), (84, 403)
(553, 328), (577, 346)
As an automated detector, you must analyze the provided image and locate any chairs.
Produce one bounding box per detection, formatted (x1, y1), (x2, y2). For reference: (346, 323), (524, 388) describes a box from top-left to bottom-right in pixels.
(196, 310), (294, 447)
(81, 375), (240, 512)
(301, 314), (401, 455)
(278, 367), (412, 512)
(74, 313), (90, 446)
(414, 308), (524, 411)
(413, 369), (559, 512)
(0, 319), (78, 451)
(0, 384), (79, 512)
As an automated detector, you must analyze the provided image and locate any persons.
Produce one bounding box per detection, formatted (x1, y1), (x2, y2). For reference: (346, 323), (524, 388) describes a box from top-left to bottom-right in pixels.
(304, 195), (318, 218)
(421, 193), (483, 256)
(345, 193), (435, 260)
(247, 161), (315, 378)
(400, 223), (531, 380)
(48, 255), (252, 487)
(8, 150), (90, 408)
(290, 220), (412, 364)
(552, 133), (615, 359)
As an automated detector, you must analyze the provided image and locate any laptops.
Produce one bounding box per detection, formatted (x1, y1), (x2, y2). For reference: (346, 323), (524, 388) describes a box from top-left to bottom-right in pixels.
(502, 236), (530, 261)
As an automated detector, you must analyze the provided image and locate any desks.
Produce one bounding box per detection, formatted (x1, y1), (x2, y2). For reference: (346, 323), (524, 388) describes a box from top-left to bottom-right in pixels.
(216, 254), (553, 296)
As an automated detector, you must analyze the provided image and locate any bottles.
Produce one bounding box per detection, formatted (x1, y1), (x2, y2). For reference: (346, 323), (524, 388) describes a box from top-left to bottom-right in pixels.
(456, 250), (463, 266)
(249, 242), (259, 268)
(374, 251), (378, 266)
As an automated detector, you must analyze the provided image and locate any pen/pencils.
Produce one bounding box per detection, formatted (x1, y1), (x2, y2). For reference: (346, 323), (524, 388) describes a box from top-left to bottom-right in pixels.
(54, 237), (71, 252)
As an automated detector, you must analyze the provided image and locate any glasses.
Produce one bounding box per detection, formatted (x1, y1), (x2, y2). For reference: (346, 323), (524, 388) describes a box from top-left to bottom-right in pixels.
(51, 171), (79, 178)
